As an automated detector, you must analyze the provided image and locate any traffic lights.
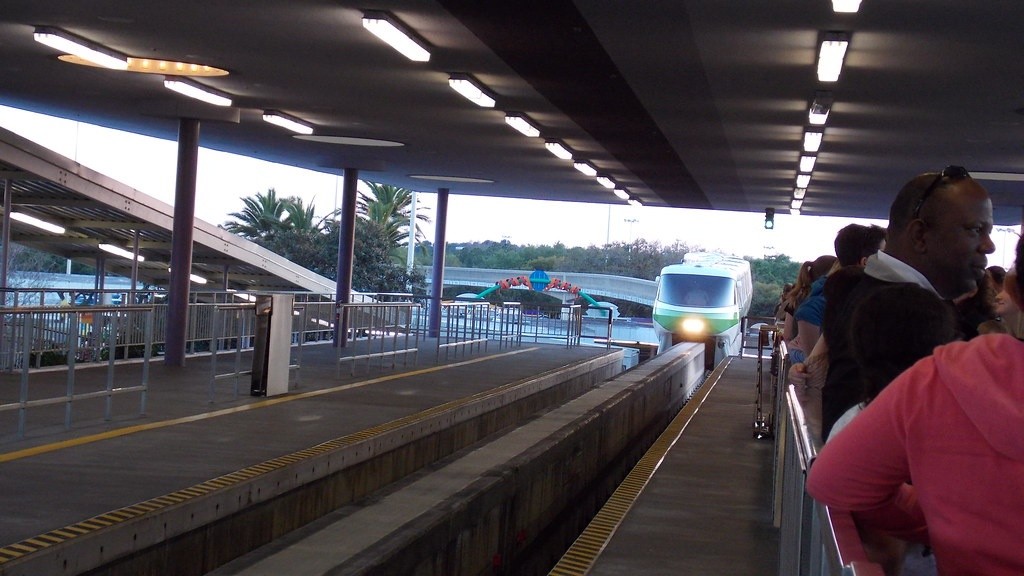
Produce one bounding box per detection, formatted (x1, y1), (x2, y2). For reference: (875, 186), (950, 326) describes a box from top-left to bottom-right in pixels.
(765, 206), (775, 228)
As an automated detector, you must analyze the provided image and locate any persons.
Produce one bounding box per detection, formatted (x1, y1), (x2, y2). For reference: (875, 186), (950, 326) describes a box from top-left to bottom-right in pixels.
(683, 282), (709, 306)
(774, 166), (1024, 576)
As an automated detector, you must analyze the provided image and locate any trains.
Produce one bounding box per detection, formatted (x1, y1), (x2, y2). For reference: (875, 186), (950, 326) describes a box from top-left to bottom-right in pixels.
(653, 251), (755, 368)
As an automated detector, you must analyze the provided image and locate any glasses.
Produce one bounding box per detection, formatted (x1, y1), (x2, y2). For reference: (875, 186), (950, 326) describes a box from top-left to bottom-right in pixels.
(914, 165), (972, 219)
